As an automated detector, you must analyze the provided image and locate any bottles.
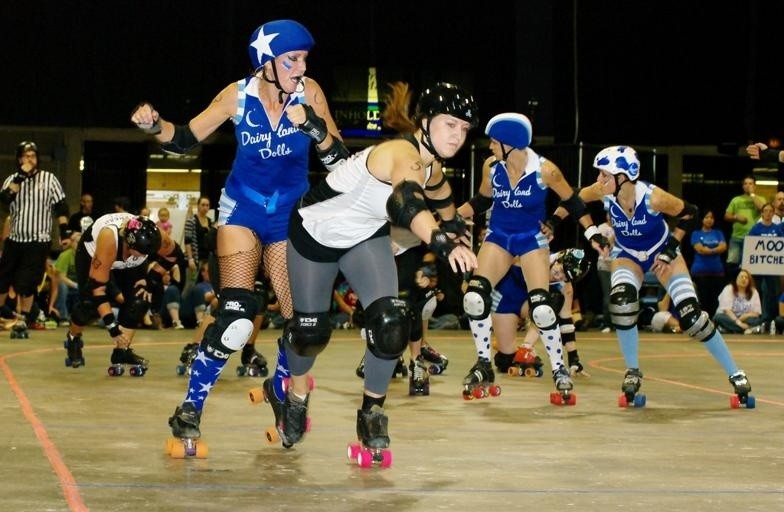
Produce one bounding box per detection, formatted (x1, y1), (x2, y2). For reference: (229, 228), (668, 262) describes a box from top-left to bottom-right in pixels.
(770, 320), (775, 334)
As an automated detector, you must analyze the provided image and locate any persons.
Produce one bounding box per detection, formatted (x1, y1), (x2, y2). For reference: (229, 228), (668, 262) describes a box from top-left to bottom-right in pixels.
(334, 224), (472, 387)
(275, 82), (480, 448)
(131, 19), (350, 446)
(572, 146), (753, 392)
(491, 139), (783, 378)
(1, 139), (282, 377)
(455, 113), (578, 388)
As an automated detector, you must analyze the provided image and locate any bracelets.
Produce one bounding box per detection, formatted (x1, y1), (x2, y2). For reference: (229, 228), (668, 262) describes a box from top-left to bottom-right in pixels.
(297, 103), (327, 143)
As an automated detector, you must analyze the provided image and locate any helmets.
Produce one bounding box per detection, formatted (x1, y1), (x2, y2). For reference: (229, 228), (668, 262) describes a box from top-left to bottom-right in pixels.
(124, 216), (162, 256)
(562, 247), (591, 284)
(248, 19), (316, 72)
(15, 140), (38, 159)
(592, 144), (641, 183)
(484, 112), (534, 150)
(414, 82), (480, 129)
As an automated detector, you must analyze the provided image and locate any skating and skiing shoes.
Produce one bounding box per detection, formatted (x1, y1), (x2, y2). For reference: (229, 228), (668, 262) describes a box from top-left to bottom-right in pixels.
(419, 344), (449, 375)
(727, 368), (756, 409)
(107, 347), (151, 377)
(407, 354), (430, 396)
(277, 373), (317, 444)
(164, 406), (210, 460)
(175, 343), (200, 376)
(462, 356), (502, 401)
(391, 354), (408, 379)
(617, 367), (647, 408)
(506, 345), (545, 377)
(63, 330), (85, 369)
(235, 349), (269, 377)
(247, 376), (294, 450)
(549, 364), (577, 405)
(9, 319), (29, 339)
(345, 404), (392, 469)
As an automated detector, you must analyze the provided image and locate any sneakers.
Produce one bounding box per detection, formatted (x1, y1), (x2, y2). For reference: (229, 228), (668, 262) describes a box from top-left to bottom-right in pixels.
(749, 325), (761, 334)
(172, 319), (185, 330)
(59, 319), (70, 327)
